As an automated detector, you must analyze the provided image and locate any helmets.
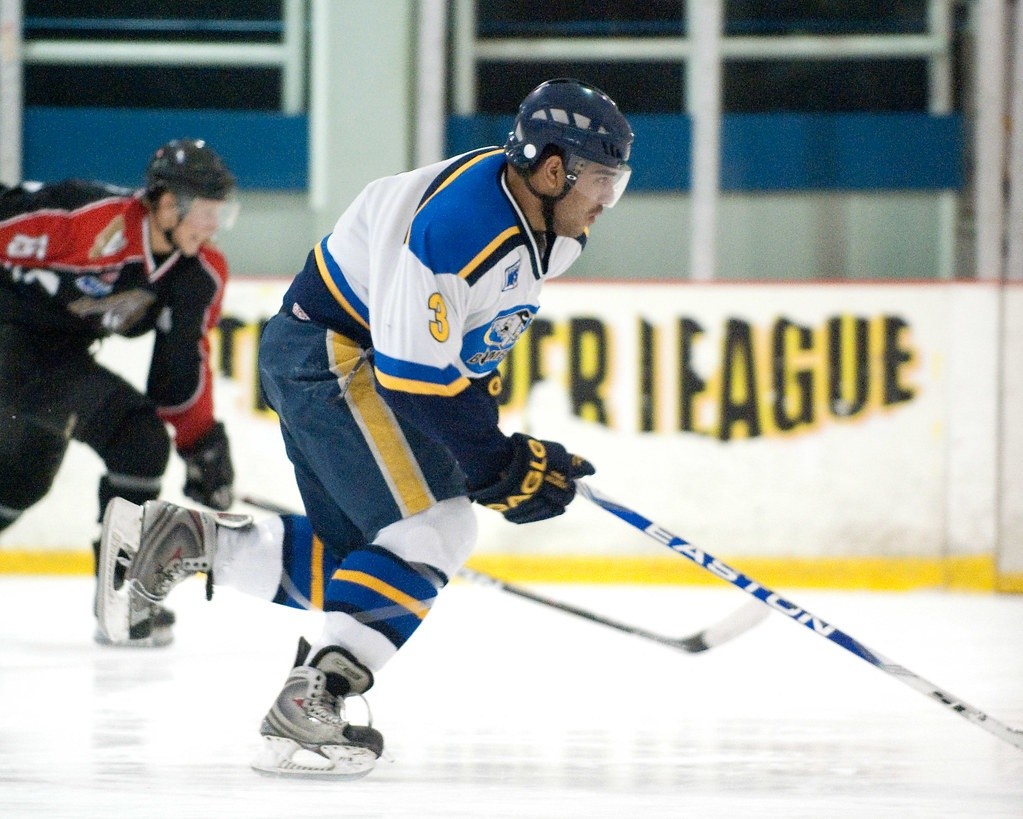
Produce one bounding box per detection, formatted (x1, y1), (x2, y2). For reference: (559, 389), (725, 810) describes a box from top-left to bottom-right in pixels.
(504, 78), (634, 210)
(147, 138), (235, 201)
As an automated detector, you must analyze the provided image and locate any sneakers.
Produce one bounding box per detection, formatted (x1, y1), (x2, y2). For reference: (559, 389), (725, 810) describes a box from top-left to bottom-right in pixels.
(237, 635), (386, 781)
(93, 496), (253, 649)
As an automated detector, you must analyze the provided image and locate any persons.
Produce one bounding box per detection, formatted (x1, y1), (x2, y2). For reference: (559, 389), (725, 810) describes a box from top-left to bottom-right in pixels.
(0, 137), (235, 646)
(97, 78), (635, 778)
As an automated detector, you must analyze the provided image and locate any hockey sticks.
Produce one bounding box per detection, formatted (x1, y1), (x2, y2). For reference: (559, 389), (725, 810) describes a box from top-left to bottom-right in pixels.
(574, 477), (1023, 749)
(233, 490), (770, 654)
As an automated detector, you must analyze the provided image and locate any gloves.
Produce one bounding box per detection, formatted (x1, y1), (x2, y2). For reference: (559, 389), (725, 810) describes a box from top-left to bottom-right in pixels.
(468, 432), (596, 524)
(465, 367), (503, 428)
(34, 315), (86, 365)
(175, 422), (235, 511)
